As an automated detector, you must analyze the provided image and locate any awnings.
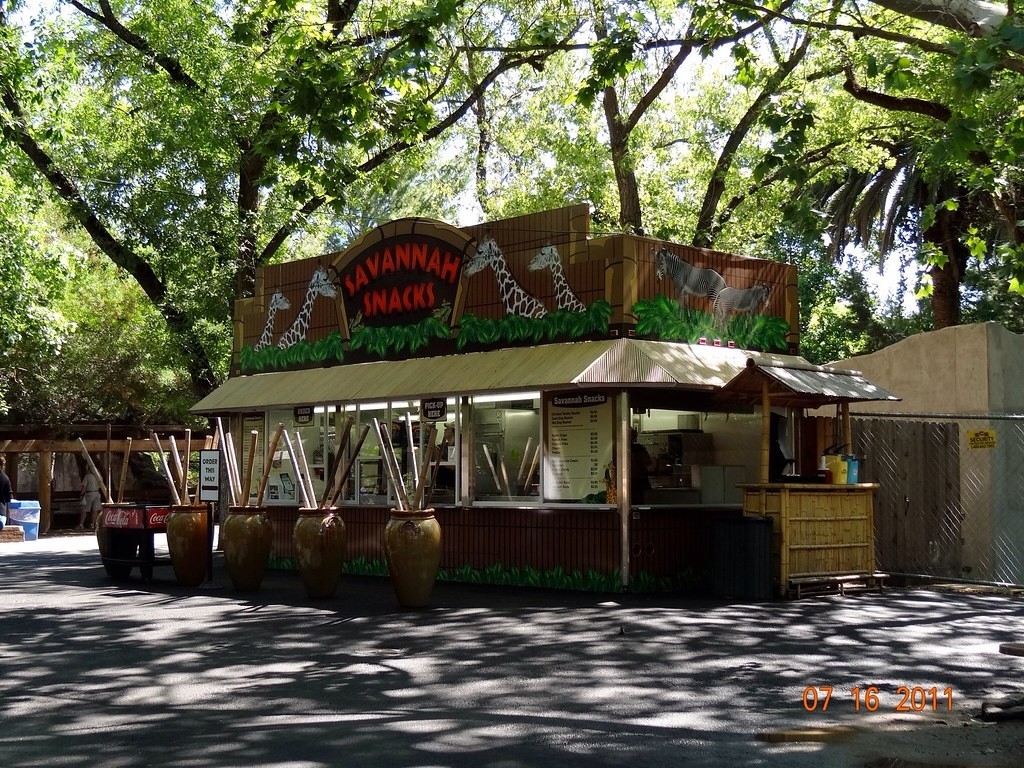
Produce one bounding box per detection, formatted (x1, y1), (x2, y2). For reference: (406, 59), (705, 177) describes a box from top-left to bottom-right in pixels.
(714, 358), (903, 484)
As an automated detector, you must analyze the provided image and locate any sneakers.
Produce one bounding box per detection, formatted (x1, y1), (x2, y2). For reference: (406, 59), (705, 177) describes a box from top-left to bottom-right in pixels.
(87, 523), (95, 529)
(73, 524), (84, 530)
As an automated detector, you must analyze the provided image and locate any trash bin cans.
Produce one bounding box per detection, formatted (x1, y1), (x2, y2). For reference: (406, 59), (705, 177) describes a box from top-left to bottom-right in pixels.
(712, 516), (775, 605)
(7, 499), (42, 541)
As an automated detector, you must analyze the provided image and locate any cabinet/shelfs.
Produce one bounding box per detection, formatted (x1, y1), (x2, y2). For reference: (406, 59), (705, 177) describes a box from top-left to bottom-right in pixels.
(637, 432), (748, 505)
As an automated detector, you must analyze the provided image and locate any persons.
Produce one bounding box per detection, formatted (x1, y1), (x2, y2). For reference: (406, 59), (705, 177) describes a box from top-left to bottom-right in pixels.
(629, 427), (656, 505)
(0, 455), (14, 533)
(73, 465), (102, 530)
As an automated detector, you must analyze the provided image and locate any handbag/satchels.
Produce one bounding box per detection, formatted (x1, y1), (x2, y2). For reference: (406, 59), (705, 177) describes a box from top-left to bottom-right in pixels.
(79, 495), (86, 506)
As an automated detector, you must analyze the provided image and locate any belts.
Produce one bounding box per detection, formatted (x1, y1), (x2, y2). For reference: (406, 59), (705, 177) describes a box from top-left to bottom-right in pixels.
(85, 490), (99, 493)
